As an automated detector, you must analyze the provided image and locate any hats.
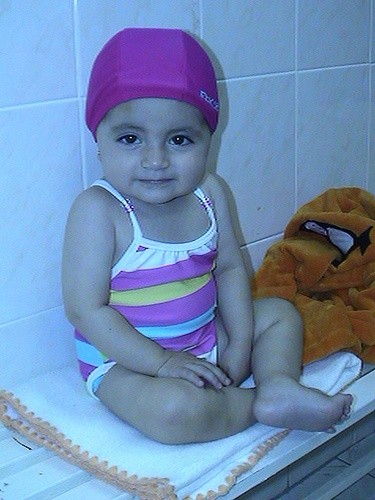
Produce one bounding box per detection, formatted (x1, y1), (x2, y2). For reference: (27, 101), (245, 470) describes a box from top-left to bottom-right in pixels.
(85, 28), (219, 143)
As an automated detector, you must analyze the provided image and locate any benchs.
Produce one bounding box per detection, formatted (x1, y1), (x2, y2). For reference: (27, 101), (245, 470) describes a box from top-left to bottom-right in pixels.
(0, 364), (375, 500)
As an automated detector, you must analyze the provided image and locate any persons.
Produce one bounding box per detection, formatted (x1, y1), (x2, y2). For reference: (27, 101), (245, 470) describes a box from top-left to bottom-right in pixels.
(63, 28), (354, 445)
